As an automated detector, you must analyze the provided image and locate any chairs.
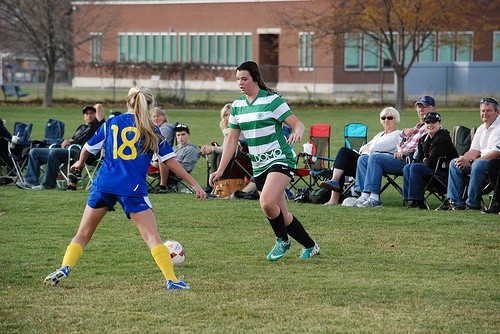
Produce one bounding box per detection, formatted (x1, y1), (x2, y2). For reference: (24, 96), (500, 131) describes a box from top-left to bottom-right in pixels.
(148, 152), (200, 195)
(286, 123), (368, 195)
(200, 139), (251, 193)
(0, 119), (103, 191)
(424, 123), (475, 211)
(379, 174), (403, 197)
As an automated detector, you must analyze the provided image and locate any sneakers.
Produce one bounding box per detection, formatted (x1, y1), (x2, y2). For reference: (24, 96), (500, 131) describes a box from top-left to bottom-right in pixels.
(266, 238), (291, 261)
(32, 185), (46, 190)
(68, 171), (82, 179)
(16, 182), (33, 188)
(299, 242), (321, 259)
(62, 185), (77, 191)
(321, 181), (341, 191)
(351, 197), (382, 207)
(166, 275), (191, 291)
(43, 266), (71, 286)
(152, 185), (167, 194)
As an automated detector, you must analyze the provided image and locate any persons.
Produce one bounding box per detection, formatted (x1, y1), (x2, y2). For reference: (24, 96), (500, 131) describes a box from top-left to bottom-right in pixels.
(209, 61), (320, 261)
(64, 112), (122, 190)
(200, 103), (292, 200)
(320, 96), (459, 210)
(17, 104), (106, 190)
(443, 97), (500, 214)
(146, 107), (175, 174)
(0, 119), (21, 178)
(43, 85), (206, 290)
(153, 124), (200, 193)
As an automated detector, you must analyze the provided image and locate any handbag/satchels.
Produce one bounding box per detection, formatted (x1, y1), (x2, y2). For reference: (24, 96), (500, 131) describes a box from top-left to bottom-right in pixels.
(214, 179), (245, 198)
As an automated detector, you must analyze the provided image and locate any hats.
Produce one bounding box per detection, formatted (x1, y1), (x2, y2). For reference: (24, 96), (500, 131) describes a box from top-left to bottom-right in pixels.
(415, 96), (436, 107)
(83, 106), (96, 114)
(173, 124), (190, 134)
(424, 112), (442, 122)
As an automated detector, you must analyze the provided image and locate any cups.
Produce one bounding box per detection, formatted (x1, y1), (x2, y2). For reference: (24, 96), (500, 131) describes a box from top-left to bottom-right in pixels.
(10, 135), (20, 148)
(303, 142), (313, 161)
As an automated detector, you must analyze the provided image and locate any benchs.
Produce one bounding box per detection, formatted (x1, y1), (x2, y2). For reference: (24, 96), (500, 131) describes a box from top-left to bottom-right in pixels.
(2, 85), (29, 103)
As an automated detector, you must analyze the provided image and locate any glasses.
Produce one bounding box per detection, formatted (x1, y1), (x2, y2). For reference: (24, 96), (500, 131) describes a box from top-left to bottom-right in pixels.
(425, 121), (438, 124)
(381, 116), (394, 120)
(481, 98), (495, 102)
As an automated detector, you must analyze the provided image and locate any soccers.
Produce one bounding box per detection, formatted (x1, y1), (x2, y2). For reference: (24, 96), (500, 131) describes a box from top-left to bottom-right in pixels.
(162, 240), (187, 265)
